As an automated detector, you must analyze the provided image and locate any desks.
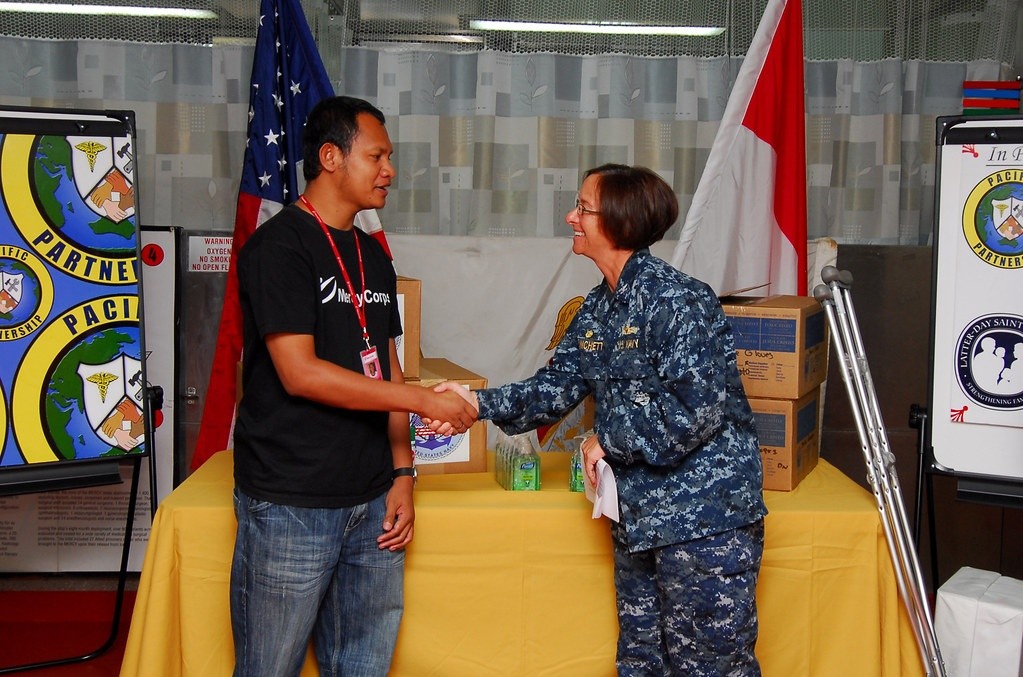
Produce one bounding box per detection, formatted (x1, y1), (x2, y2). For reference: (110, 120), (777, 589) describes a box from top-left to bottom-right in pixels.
(117, 448), (926, 677)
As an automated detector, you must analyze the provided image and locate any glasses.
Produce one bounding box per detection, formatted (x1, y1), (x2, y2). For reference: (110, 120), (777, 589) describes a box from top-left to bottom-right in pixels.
(575, 199), (601, 216)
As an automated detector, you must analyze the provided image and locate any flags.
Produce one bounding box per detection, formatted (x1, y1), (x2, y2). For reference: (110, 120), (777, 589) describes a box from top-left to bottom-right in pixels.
(670, 0), (808, 298)
(188, 0), (394, 470)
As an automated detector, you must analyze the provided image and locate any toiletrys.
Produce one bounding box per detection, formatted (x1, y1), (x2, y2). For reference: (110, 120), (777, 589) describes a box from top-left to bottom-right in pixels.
(494, 425), (541, 491)
(569, 434), (588, 492)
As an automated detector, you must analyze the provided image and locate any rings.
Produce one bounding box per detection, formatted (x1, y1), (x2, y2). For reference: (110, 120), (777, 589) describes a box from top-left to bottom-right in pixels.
(455, 423), (463, 429)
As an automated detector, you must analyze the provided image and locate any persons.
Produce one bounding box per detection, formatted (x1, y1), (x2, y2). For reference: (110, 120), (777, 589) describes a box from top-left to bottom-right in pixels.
(418, 162), (769, 677)
(223, 96), (478, 677)
(369, 363), (377, 377)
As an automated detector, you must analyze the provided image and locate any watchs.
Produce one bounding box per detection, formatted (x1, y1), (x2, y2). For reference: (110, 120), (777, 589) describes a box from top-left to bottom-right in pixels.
(391, 467), (418, 483)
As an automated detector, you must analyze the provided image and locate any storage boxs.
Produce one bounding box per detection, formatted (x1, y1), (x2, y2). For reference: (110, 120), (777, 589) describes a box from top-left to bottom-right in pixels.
(716, 294), (830, 400)
(744, 384), (822, 492)
(392, 274), (423, 381)
(402, 356), (489, 473)
